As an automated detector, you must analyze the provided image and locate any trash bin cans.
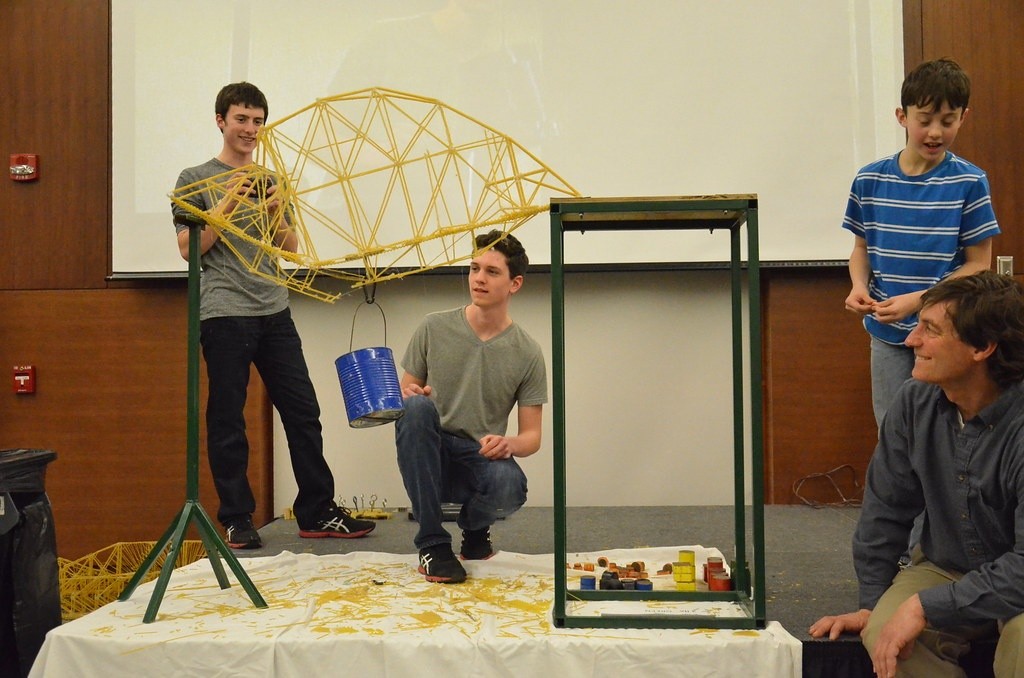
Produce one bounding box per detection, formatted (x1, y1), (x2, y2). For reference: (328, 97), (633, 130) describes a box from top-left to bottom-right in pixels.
(0, 450), (64, 678)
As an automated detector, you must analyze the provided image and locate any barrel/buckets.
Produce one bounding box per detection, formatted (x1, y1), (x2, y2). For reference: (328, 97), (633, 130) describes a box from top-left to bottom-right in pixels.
(336, 301), (405, 428)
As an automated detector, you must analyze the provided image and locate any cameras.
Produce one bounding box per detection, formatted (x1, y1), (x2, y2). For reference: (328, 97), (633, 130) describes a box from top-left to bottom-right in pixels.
(245, 177), (272, 198)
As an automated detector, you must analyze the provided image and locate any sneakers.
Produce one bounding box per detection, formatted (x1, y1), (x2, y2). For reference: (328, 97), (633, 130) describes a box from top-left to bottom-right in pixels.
(225, 516), (261, 548)
(299, 506), (376, 538)
(459, 525), (495, 560)
(418, 542), (467, 583)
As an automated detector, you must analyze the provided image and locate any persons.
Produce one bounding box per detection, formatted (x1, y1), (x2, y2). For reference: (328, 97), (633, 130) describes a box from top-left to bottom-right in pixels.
(393, 228), (549, 585)
(809, 271), (1023, 678)
(842, 55), (1001, 572)
(172, 81), (377, 551)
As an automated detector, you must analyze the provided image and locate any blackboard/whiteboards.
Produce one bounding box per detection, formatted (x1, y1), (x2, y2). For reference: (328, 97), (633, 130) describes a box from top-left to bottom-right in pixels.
(101, 1), (906, 282)
